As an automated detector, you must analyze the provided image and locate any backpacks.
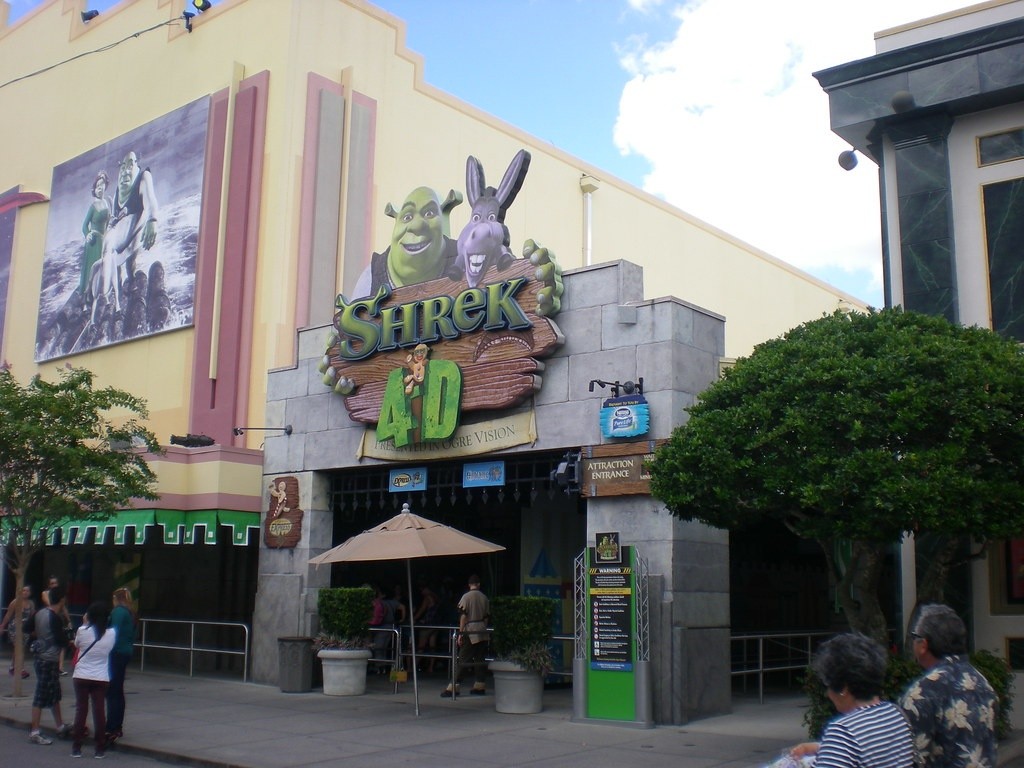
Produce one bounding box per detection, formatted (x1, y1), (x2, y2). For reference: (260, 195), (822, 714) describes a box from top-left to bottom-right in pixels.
(372, 599), (385, 625)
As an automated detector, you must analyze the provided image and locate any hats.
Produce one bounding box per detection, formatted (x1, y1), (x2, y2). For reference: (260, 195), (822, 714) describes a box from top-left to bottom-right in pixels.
(469, 575), (480, 583)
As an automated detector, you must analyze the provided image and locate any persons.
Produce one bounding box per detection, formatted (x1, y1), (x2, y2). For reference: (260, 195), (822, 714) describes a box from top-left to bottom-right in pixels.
(896, 603), (999, 768)
(70, 602), (116, 759)
(365, 581), (439, 676)
(790, 633), (914, 768)
(106, 588), (136, 746)
(0, 585), (34, 679)
(28, 587), (73, 745)
(42, 575), (72, 675)
(439, 575), (490, 697)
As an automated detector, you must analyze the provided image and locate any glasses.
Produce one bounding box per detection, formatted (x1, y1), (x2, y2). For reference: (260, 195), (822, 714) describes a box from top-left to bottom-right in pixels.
(911, 632), (922, 640)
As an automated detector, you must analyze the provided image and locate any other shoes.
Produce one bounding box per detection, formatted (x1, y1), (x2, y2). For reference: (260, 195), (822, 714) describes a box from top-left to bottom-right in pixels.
(107, 735), (119, 745)
(376, 668), (386, 674)
(116, 726), (124, 736)
(59, 670), (67, 676)
(9, 667), (15, 675)
(94, 752), (106, 758)
(70, 751), (83, 757)
(21, 669), (29, 679)
(441, 690), (459, 697)
(470, 689), (485, 695)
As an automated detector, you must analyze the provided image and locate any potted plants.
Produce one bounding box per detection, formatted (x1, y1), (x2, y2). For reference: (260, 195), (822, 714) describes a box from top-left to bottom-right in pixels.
(484, 595), (560, 713)
(312, 587), (379, 695)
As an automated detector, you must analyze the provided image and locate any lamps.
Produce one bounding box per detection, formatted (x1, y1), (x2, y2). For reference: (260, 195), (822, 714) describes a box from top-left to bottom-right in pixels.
(890, 90), (915, 112)
(193, 0), (212, 10)
(589, 377), (643, 397)
(838, 148), (859, 170)
(231, 425), (292, 435)
(167, 433), (215, 448)
(183, 11), (195, 32)
(80, 10), (97, 22)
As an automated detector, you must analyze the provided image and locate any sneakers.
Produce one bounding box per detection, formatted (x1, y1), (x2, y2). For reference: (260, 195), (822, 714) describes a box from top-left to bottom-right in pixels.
(25, 729), (53, 745)
(57, 724), (73, 737)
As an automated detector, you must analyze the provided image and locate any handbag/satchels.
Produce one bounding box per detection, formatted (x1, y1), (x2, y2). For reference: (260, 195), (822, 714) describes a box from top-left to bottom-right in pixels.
(30, 639), (46, 653)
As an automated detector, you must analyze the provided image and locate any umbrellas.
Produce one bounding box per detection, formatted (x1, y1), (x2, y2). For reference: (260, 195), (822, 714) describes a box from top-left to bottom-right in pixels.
(307, 503), (506, 716)
